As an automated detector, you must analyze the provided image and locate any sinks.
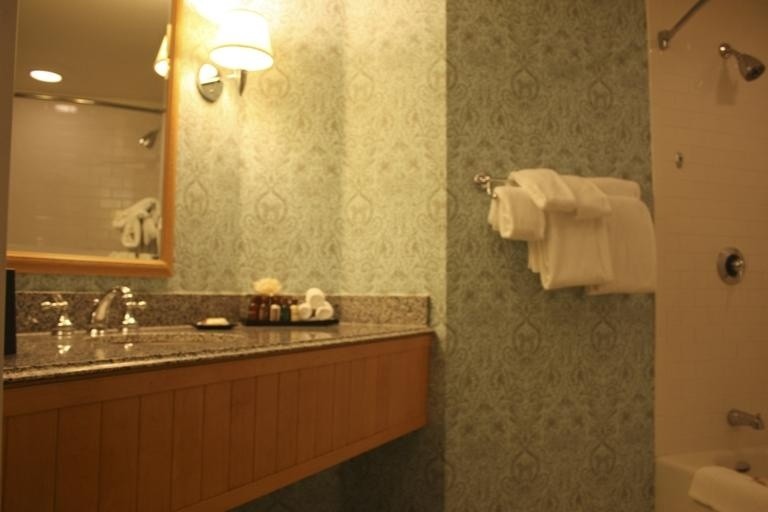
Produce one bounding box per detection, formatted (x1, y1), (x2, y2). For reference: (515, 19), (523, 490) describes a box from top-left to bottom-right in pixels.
(88, 334), (235, 346)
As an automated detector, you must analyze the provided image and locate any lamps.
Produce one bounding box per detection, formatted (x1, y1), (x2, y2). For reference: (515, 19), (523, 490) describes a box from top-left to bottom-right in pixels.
(196, 7), (274, 104)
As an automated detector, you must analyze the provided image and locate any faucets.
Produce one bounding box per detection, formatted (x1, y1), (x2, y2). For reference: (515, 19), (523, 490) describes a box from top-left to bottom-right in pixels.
(728, 410), (764, 433)
(90, 286), (144, 330)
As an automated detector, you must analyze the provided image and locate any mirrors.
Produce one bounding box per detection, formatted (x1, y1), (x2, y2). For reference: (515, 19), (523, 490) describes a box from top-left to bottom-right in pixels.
(0, 0), (178, 279)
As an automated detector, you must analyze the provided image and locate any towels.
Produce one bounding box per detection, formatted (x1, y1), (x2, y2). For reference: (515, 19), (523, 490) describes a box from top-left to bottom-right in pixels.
(107, 196), (162, 259)
(688, 464), (767, 510)
(487, 167), (660, 296)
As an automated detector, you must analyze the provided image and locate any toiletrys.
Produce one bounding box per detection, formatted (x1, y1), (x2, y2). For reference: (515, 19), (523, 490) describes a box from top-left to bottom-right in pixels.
(247, 296), (298, 322)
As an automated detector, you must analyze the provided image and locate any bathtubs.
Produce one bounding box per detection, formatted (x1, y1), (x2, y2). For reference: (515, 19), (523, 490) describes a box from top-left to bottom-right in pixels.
(653, 445), (767, 511)
